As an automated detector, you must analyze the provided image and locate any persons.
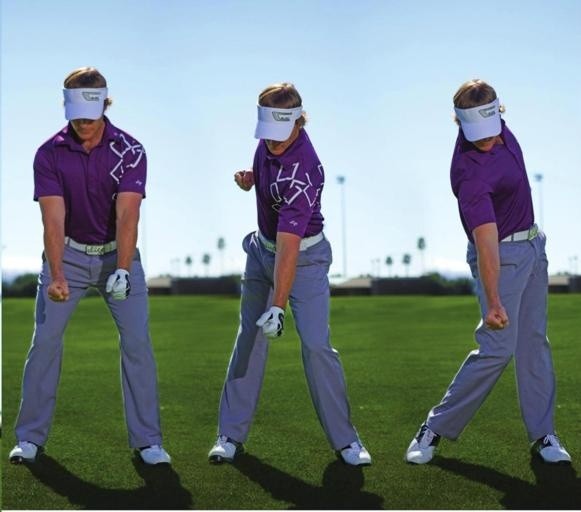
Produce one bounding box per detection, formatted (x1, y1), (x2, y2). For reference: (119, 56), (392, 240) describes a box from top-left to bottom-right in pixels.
(207, 81), (372, 466)
(405, 79), (572, 464)
(9, 69), (171, 466)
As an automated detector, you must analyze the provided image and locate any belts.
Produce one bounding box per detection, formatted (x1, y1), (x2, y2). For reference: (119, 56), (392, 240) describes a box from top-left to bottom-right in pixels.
(63, 237), (117, 255)
(500, 224), (538, 243)
(257, 227), (323, 255)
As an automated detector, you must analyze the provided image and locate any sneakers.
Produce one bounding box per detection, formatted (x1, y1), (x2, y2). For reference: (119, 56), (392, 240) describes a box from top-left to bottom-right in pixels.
(136, 444), (171, 465)
(9, 441), (38, 464)
(404, 421), (440, 465)
(536, 434), (572, 463)
(208, 436), (244, 463)
(340, 440), (372, 466)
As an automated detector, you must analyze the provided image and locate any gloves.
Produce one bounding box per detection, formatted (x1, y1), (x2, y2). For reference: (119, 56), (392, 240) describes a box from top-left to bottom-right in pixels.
(256, 306), (285, 339)
(106, 269), (132, 301)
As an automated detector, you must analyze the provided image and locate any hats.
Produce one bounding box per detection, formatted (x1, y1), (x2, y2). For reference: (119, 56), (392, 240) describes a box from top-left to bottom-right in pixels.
(254, 105), (301, 142)
(455, 97), (502, 142)
(63, 88), (108, 121)
